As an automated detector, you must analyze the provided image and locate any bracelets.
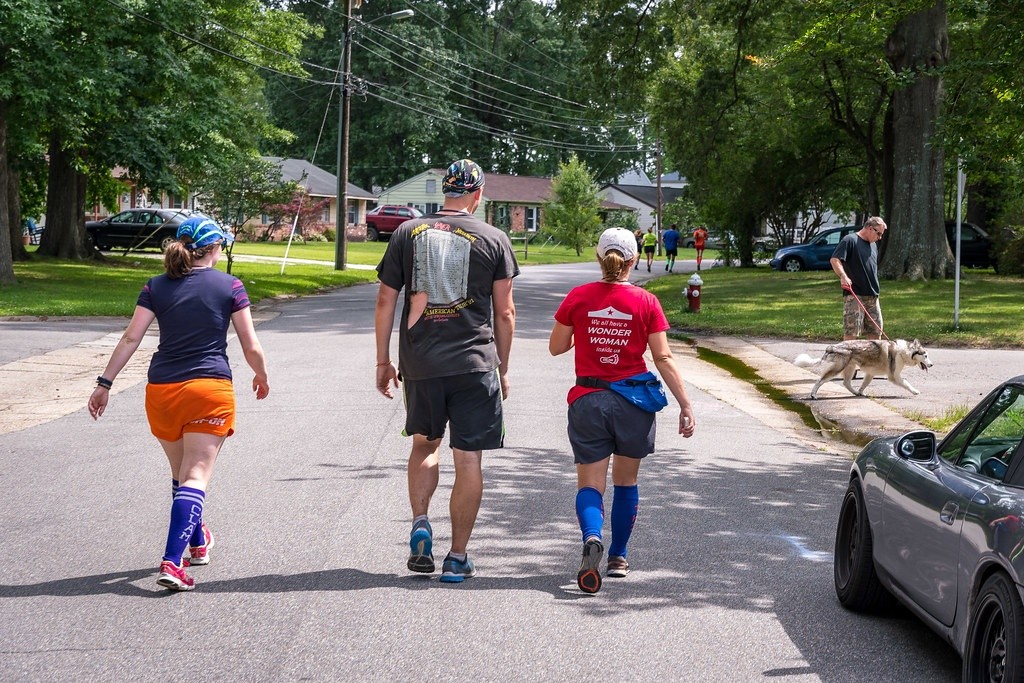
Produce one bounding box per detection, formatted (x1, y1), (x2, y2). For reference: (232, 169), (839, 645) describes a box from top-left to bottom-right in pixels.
(98, 384), (110, 390)
(97, 376), (112, 387)
(377, 361), (391, 366)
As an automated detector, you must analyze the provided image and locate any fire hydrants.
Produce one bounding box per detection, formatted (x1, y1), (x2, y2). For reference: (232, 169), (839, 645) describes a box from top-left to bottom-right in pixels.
(681, 272), (704, 314)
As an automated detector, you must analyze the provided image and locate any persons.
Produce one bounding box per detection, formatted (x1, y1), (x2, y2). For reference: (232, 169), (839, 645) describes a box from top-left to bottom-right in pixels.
(88, 216), (269, 589)
(633, 230), (642, 270)
(550, 228), (695, 593)
(376, 159), (520, 582)
(831, 216), (888, 378)
(662, 224), (682, 273)
(641, 228), (657, 272)
(694, 225), (709, 270)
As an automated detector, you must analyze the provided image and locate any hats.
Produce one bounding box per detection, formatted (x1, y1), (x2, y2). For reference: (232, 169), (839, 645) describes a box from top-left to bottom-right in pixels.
(176, 217), (234, 249)
(441, 159), (485, 197)
(597, 227), (639, 261)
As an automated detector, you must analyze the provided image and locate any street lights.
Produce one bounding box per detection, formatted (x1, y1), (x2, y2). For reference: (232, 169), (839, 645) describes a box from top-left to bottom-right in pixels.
(334, 7), (417, 271)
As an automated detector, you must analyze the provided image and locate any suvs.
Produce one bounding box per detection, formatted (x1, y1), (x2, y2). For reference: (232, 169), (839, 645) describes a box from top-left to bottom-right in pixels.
(752, 228), (807, 254)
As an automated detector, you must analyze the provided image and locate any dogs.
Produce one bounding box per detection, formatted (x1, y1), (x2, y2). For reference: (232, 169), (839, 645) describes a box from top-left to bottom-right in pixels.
(792, 337), (934, 401)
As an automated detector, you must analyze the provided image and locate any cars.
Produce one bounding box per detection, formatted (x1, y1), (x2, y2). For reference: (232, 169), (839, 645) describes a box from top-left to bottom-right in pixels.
(166, 208), (210, 222)
(681, 230), (735, 249)
(944, 220), (1000, 274)
(835, 374), (1024, 683)
(84, 208), (189, 254)
(769, 226), (863, 273)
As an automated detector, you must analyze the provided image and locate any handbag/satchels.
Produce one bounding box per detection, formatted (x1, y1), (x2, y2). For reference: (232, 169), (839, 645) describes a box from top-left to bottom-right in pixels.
(576, 370), (668, 413)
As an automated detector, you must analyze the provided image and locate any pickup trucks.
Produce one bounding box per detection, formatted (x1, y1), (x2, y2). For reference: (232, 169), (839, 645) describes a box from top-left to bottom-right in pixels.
(366, 205), (426, 242)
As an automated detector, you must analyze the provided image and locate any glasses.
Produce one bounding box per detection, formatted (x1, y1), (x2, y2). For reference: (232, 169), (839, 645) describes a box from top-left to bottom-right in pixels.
(872, 227), (883, 237)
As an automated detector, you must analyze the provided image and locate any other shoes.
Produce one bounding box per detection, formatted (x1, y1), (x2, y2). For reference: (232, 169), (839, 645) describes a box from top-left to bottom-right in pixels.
(635, 267), (638, 270)
(665, 265), (668, 271)
(647, 265), (650, 272)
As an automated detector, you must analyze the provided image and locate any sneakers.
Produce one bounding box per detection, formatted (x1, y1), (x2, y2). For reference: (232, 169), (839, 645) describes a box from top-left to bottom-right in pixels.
(189, 523), (214, 564)
(577, 537), (603, 593)
(606, 555), (631, 576)
(156, 558), (195, 591)
(407, 519), (435, 573)
(440, 552), (477, 582)
